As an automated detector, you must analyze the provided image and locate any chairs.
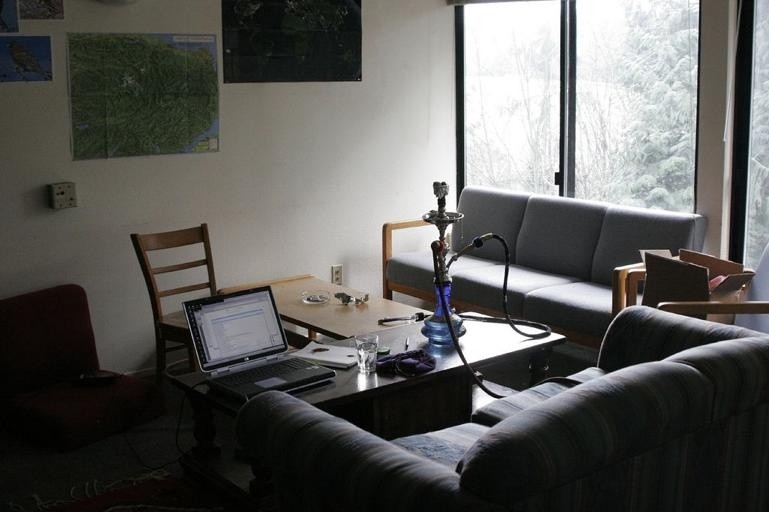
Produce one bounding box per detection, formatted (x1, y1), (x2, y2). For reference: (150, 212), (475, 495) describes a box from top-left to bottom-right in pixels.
(131, 222), (215, 373)
(0, 283), (165, 451)
(627, 243), (769, 333)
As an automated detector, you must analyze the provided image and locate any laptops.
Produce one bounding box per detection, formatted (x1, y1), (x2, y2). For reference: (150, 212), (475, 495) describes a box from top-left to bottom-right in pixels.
(181, 283), (338, 402)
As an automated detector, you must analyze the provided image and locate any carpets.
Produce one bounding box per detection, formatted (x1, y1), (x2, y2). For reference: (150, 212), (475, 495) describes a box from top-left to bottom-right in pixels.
(7, 467), (255, 512)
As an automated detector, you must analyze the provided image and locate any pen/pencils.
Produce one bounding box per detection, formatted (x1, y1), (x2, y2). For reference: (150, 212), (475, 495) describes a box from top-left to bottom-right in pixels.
(406, 336), (409, 350)
(347, 355), (355, 357)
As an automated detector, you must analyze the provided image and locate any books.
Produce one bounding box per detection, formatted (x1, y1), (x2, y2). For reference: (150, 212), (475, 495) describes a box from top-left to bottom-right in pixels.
(288, 340), (359, 369)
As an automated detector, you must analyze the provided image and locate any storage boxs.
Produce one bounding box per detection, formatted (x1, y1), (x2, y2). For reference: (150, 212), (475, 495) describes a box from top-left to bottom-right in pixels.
(638, 250), (756, 325)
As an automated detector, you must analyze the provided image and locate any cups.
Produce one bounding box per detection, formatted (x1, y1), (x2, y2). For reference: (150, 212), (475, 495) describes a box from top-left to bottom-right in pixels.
(355, 334), (378, 373)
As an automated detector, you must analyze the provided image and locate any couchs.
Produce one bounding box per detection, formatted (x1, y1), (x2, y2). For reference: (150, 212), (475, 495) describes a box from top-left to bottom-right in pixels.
(384, 186), (707, 368)
(235, 305), (769, 512)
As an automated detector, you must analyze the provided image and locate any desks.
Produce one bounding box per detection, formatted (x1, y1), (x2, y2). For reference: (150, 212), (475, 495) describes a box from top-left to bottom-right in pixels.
(169, 275), (565, 478)
